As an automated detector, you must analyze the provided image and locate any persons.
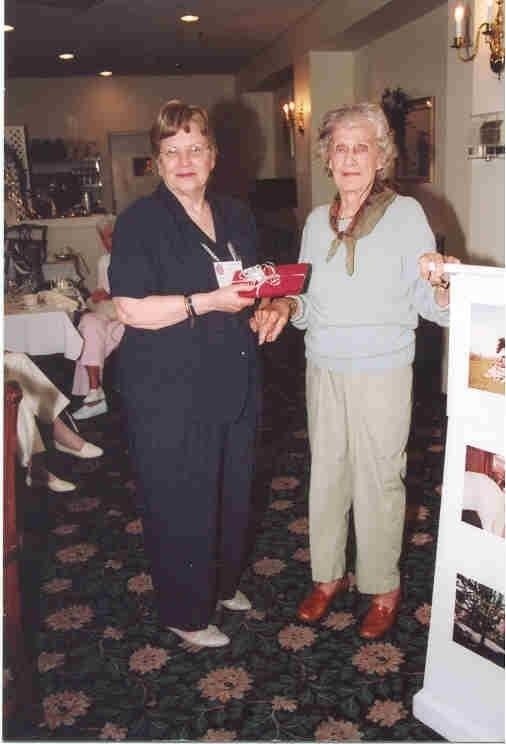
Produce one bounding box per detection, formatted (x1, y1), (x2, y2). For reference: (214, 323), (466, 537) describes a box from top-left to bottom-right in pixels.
(482, 336), (505, 382)
(107, 97), (280, 650)
(4, 350), (106, 494)
(249, 101), (462, 639)
(72, 213), (125, 420)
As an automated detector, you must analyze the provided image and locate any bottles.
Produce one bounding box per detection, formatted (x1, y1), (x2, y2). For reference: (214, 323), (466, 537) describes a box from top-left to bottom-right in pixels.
(81, 191), (92, 216)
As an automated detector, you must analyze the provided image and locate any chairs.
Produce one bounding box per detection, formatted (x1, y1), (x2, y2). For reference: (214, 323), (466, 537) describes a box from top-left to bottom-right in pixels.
(5, 224), (48, 293)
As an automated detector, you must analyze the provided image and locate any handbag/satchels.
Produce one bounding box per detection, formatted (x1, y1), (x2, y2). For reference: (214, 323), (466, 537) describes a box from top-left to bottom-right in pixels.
(87, 298), (118, 320)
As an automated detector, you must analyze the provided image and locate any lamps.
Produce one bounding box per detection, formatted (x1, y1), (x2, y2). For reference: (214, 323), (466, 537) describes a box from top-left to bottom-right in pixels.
(283, 101), (304, 135)
(449, 0), (504, 73)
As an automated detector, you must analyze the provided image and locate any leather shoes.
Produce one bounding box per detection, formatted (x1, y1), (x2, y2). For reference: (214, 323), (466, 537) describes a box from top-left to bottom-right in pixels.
(360, 591), (400, 638)
(72, 400), (108, 420)
(54, 441), (104, 459)
(166, 624), (231, 648)
(83, 389), (105, 403)
(26, 476), (76, 493)
(296, 580), (343, 622)
(218, 589), (252, 611)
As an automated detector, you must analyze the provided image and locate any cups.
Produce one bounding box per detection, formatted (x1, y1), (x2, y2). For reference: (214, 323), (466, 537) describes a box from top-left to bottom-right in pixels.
(24, 295), (39, 305)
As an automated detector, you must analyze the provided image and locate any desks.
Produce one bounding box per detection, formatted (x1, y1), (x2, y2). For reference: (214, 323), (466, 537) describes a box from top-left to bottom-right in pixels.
(21, 215), (118, 297)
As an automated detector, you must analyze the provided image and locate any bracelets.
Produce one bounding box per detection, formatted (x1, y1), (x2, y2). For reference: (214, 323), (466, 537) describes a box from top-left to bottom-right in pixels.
(184, 291), (197, 321)
(282, 294), (298, 320)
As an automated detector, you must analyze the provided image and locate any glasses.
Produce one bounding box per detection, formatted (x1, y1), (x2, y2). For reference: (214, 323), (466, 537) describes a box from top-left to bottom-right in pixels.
(158, 146), (211, 157)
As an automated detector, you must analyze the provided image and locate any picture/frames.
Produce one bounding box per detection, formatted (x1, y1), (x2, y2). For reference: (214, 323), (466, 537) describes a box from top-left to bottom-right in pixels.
(393, 96), (434, 184)
